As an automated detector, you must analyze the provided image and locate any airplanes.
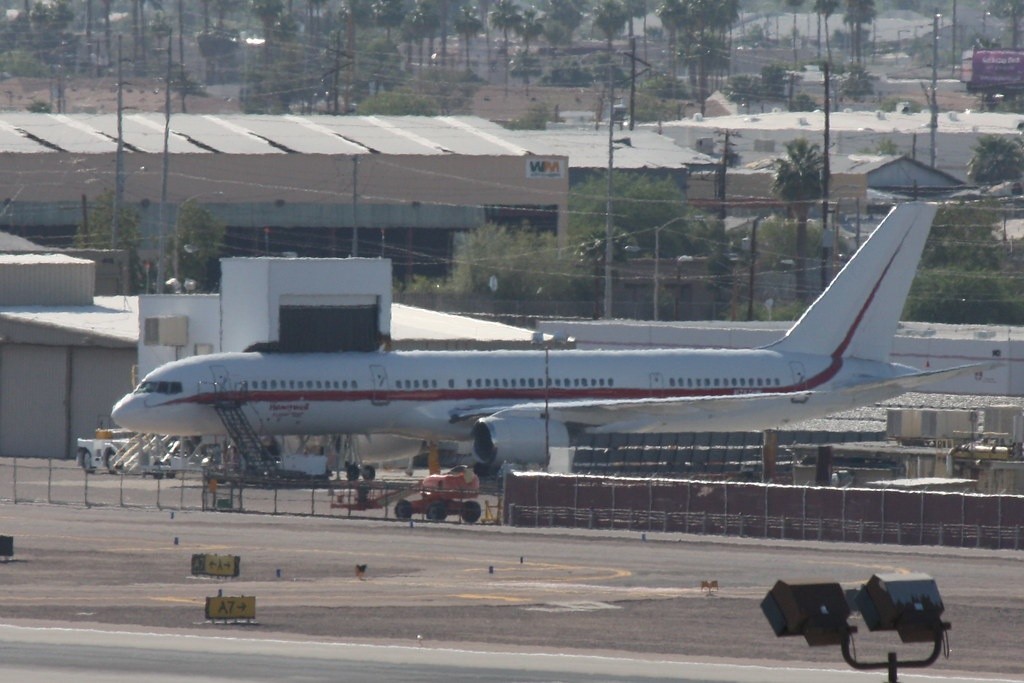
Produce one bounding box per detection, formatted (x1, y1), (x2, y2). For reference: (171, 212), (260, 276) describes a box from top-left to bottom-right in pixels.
(110, 201), (1008, 482)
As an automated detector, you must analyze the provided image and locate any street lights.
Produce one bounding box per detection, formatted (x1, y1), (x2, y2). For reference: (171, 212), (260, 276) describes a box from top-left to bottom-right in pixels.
(760, 575), (952, 682)
(530, 332), (577, 470)
